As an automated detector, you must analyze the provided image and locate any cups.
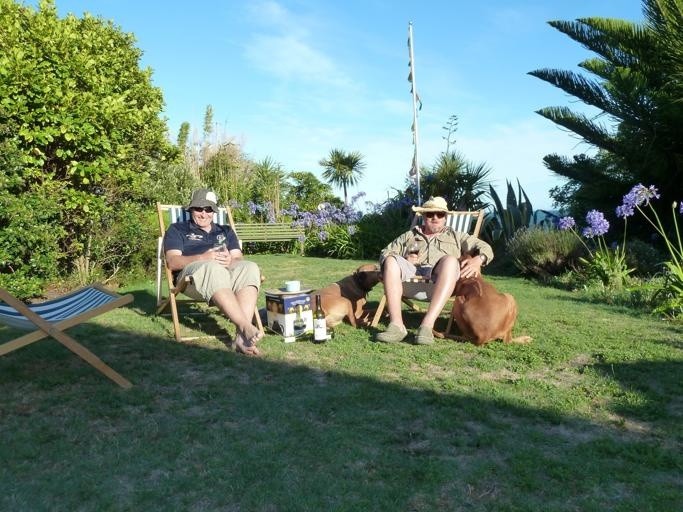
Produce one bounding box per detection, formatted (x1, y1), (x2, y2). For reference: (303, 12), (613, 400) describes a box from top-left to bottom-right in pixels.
(409, 246), (419, 254)
(213, 243), (224, 252)
(285, 281), (301, 292)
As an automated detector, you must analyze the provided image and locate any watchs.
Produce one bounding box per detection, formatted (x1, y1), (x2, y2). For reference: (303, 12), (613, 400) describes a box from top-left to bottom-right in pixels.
(479, 252), (487, 263)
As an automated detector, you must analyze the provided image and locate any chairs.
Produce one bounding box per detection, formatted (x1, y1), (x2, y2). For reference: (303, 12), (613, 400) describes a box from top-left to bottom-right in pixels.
(234, 222), (305, 257)
(0, 279), (136, 390)
(371, 206), (485, 339)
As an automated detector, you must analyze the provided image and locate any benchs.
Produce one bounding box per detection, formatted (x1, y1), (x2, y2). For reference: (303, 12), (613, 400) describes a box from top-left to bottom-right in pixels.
(152, 200), (266, 344)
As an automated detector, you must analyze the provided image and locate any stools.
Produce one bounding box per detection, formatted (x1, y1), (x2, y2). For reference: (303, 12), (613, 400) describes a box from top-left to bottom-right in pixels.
(265, 293), (313, 343)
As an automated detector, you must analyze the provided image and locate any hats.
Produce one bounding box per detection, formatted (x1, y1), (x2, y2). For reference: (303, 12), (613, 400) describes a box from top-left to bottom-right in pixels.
(412, 197), (456, 216)
(185, 189), (220, 213)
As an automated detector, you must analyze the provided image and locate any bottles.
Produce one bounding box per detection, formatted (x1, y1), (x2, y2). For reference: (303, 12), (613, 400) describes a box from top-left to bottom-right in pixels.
(313, 294), (327, 344)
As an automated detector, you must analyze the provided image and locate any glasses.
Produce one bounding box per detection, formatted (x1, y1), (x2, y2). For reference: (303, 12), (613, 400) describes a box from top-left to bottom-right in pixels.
(424, 211), (447, 218)
(194, 207), (214, 213)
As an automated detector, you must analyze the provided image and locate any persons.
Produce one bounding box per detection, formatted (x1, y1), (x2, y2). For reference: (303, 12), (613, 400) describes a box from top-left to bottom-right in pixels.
(375, 196), (495, 344)
(164, 189), (265, 356)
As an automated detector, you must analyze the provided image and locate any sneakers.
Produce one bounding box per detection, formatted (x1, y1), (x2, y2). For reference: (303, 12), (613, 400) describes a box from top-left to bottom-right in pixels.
(374, 324), (409, 343)
(415, 325), (435, 346)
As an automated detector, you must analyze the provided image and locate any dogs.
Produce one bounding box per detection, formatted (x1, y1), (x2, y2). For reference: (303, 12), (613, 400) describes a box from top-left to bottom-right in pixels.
(449, 276), (535, 347)
(309, 263), (388, 329)
